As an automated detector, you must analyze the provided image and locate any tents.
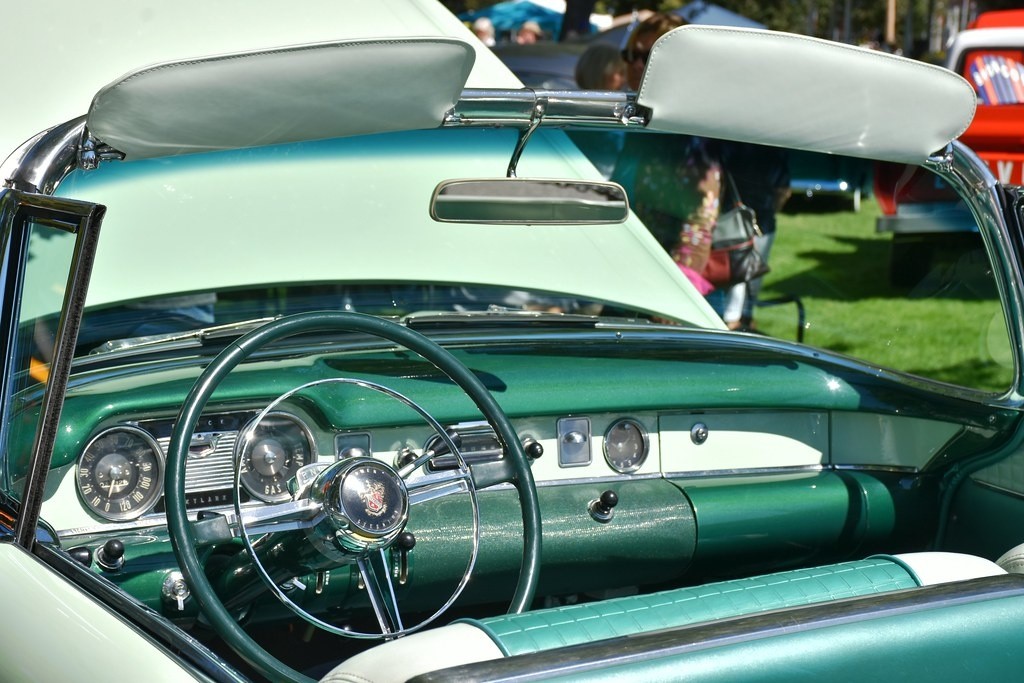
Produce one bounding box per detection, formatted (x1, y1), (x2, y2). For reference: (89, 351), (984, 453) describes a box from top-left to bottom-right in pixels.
(445, 0), (602, 48)
(583, 3), (765, 53)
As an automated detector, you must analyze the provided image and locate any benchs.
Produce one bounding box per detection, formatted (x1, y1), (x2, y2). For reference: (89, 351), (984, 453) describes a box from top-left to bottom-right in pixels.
(312, 549), (1024, 682)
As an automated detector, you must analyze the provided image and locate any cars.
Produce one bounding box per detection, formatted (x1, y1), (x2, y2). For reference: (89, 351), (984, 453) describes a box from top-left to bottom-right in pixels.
(873, 7), (1024, 283)
(0, 0), (1024, 683)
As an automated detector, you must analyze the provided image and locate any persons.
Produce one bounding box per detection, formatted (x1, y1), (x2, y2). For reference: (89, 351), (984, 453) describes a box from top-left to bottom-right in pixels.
(465, 10), (793, 342)
(30, 291), (217, 364)
